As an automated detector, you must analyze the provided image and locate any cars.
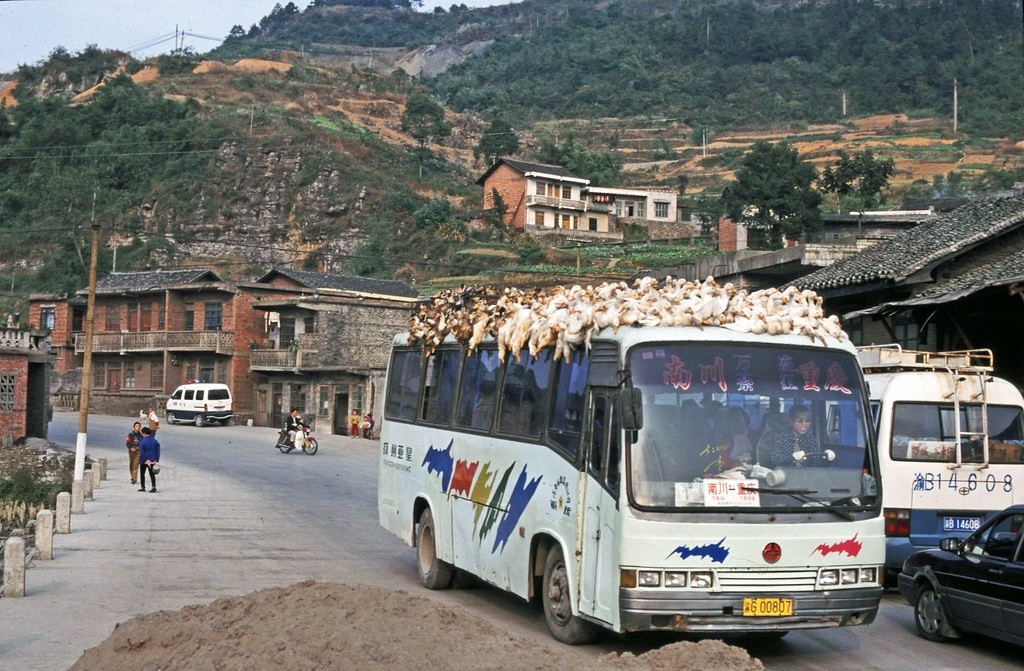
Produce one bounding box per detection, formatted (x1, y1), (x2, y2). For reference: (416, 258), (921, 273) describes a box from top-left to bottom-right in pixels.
(897, 504), (1024, 646)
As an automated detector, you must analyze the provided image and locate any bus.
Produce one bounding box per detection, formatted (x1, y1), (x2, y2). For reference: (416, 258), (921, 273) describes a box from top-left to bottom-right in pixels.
(659, 343), (1024, 592)
(376, 325), (887, 647)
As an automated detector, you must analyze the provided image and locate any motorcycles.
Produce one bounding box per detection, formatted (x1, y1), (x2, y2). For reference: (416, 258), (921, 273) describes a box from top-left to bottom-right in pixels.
(275, 417), (318, 456)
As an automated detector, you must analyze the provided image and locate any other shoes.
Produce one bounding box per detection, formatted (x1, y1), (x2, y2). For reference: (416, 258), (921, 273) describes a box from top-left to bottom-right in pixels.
(138, 488), (145, 491)
(150, 488), (156, 492)
(290, 442), (295, 448)
(132, 480), (137, 484)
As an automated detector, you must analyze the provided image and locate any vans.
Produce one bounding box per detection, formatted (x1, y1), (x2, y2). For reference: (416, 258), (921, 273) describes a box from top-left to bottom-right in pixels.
(165, 378), (235, 427)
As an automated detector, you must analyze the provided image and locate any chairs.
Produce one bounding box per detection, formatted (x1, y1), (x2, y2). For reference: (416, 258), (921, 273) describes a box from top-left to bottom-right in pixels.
(752, 412), (789, 465)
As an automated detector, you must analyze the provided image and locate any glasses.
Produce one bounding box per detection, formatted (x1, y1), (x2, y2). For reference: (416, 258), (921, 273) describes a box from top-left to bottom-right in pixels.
(791, 416), (811, 422)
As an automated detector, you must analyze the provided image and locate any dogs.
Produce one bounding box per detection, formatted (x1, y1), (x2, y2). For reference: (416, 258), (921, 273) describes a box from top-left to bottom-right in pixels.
(139, 410), (147, 423)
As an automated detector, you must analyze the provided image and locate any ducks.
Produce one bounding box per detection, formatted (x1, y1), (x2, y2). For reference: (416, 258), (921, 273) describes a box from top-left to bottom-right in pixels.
(407, 273), (849, 363)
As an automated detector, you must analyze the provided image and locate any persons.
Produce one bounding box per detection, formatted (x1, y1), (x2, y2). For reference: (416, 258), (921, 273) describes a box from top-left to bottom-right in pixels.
(359, 414), (375, 438)
(286, 408), (310, 448)
(125, 422), (143, 484)
(148, 407), (159, 438)
(351, 409), (360, 439)
(768, 405), (836, 470)
(680, 400), (758, 475)
(137, 427), (161, 492)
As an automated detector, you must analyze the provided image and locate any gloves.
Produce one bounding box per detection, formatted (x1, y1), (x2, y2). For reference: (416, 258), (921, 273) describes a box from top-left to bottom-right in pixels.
(823, 450), (835, 461)
(792, 451), (808, 461)
